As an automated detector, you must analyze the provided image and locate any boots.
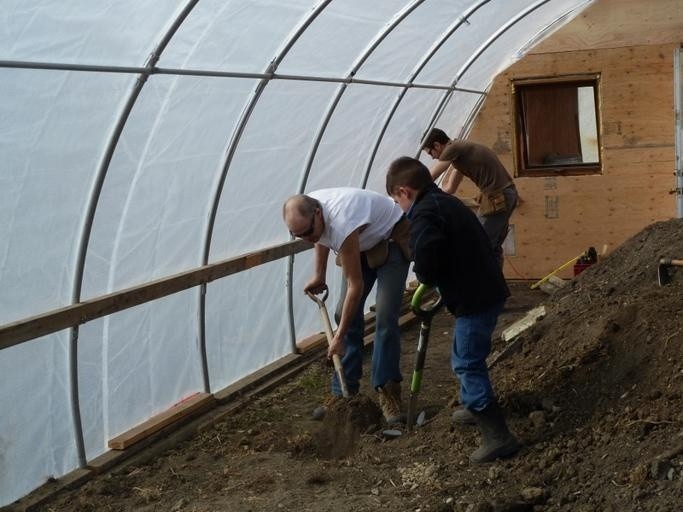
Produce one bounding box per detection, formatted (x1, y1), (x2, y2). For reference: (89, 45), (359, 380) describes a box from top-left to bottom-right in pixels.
(452, 410), (479, 424)
(470, 401), (515, 462)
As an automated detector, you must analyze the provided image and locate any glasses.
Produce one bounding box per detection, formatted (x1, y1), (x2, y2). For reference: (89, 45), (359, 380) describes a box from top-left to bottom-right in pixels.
(428, 148), (433, 156)
(289, 211), (314, 238)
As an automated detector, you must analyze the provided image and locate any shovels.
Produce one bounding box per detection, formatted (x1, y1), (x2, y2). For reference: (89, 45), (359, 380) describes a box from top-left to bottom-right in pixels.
(384, 282), (444, 437)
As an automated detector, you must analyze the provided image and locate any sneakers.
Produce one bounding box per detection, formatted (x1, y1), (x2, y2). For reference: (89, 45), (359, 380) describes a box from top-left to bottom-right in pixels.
(313, 396), (344, 419)
(378, 383), (407, 425)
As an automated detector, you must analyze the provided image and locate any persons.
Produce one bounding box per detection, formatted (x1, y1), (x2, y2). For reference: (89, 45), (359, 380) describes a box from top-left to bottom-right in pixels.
(383, 155), (521, 464)
(280, 186), (409, 427)
(421, 128), (519, 299)
